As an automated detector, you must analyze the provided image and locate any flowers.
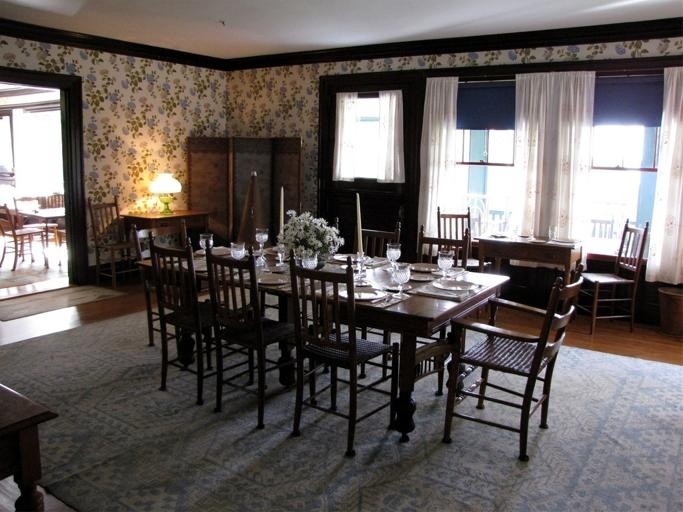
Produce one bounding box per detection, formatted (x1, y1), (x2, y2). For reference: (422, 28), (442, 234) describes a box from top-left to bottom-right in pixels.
(276, 208), (345, 261)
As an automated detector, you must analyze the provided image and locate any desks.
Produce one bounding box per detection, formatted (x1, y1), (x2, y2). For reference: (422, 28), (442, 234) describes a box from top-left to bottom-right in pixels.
(0, 380), (59, 512)
(139, 246), (510, 442)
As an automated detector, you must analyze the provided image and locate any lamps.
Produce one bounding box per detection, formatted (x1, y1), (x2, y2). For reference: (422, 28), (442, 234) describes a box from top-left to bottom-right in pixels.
(150, 173), (182, 214)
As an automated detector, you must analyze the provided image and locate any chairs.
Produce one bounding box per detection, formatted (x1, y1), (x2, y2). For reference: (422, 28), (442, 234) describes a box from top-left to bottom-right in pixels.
(441, 263), (585, 459)
(353, 221), (402, 260)
(205, 239), (304, 430)
(85, 195), (139, 288)
(1, 194), (67, 271)
(148, 231), (256, 406)
(132, 219), (191, 348)
(580, 219), (649, 336)
(416, 224), (470, 271)
(288, 247), (400, 460)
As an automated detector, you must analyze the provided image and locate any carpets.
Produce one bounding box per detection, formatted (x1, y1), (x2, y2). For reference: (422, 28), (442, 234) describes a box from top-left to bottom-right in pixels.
(0, 286), (682, 511)
(0, 284), (127, 321)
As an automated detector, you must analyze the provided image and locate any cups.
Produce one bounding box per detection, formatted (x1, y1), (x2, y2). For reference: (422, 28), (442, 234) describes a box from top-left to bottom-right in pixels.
(385, 243), (401, 269)
(229, 241), (245, 260)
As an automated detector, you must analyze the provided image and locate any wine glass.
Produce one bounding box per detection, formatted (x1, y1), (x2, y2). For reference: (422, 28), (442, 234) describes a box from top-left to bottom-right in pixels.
(253, 227), (271, 259)
(391, 263), (414, 302)
(437, 249), (455, 278)
(199, 232), (213, 261)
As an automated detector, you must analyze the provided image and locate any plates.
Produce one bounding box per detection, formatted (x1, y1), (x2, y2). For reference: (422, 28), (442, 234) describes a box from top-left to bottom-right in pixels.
(258, 274), (291, 286)
(180, 261), (218, 272)
(243, 250), (266, 257)
(194, 247), (231, 256)
(430, 280), (480, 292)
(408, 273), (437, 282)
(488, 231), (574, 244)
(342, 264), (368, 270)
(410, 261), (437, 273)
(334, 254), (359, 261)
(338, 287), (387, 302)
